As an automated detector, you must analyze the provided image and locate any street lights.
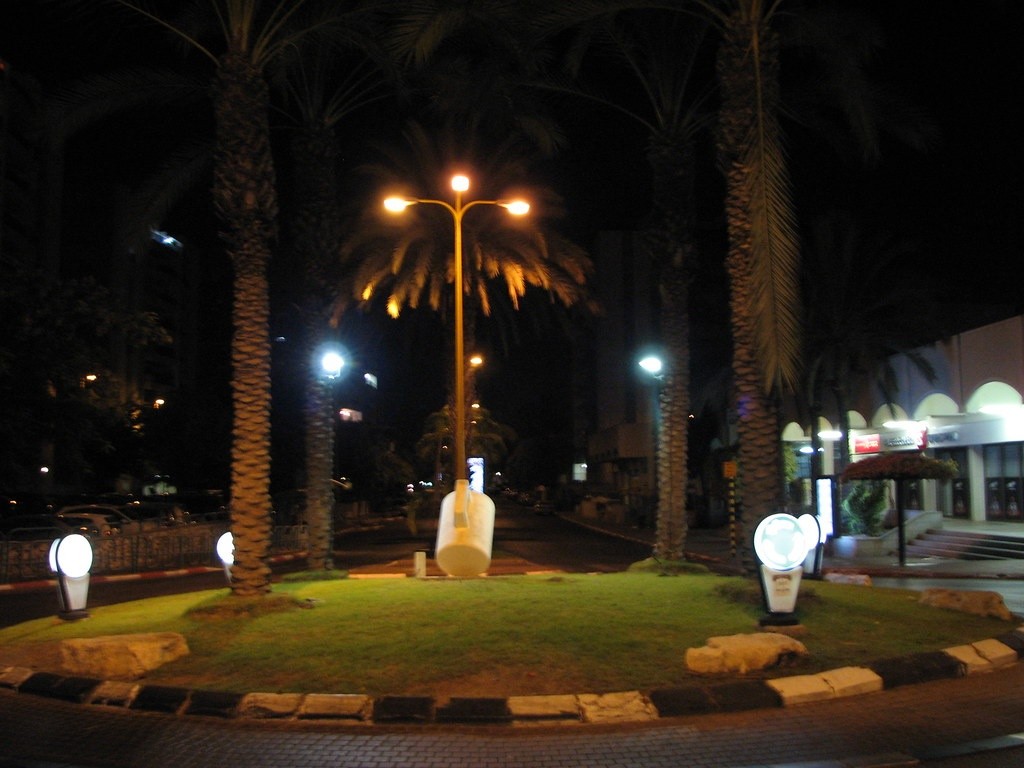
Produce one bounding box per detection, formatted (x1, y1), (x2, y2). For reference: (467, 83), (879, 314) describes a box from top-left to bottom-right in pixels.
(385, 175), (527, 480)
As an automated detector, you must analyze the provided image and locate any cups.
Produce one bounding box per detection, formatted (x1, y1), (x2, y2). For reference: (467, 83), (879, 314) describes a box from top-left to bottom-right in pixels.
(760, 564), (803, 613)
(434, 479), (496, 575)
(54, 573), (91, 610)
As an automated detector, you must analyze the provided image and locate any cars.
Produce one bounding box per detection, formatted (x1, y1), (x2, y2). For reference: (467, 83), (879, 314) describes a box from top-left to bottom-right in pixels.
(0, 492), (229, 545)
